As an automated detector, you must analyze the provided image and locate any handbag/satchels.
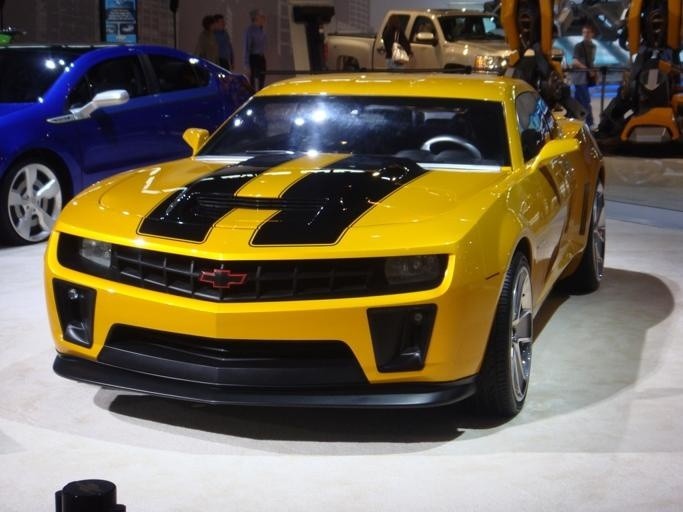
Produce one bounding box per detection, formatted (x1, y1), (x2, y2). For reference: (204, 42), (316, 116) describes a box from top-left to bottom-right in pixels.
(586, 71), (599, 87)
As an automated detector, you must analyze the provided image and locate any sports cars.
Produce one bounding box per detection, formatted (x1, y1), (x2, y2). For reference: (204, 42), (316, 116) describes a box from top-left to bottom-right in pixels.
(44, 70), (608, 426)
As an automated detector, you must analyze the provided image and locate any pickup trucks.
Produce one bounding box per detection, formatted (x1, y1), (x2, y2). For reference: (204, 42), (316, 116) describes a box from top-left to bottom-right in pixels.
(323, 8), (522, 78)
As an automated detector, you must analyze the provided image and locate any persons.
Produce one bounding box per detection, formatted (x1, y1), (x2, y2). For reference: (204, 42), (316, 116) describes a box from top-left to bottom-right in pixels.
(193, 16), (220, 67)
(241, 8), (267, 95)
(213, 14), (233, 73)
(571, 24), (598, 129)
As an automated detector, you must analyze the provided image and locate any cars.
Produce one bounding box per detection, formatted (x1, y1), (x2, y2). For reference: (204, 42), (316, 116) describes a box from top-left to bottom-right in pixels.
(0, 44), (251, 245)
